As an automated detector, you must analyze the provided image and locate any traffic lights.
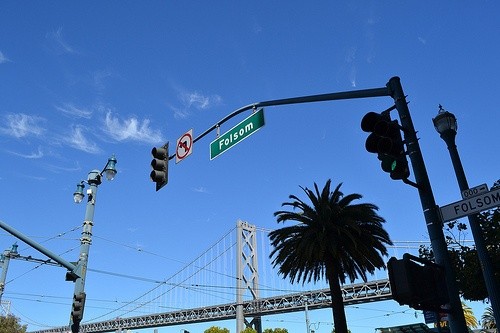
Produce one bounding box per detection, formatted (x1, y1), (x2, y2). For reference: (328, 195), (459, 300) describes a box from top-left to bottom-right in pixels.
(379, 118), (411, 181)
(69, 292), (86, 324)
(150, 140), (169, 192)
(361, 111), (393, 153)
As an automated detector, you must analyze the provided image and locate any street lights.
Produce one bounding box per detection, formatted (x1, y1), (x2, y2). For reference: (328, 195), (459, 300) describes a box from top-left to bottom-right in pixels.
(68, 152), (118, 333)
(431, 102), (500, 333)
